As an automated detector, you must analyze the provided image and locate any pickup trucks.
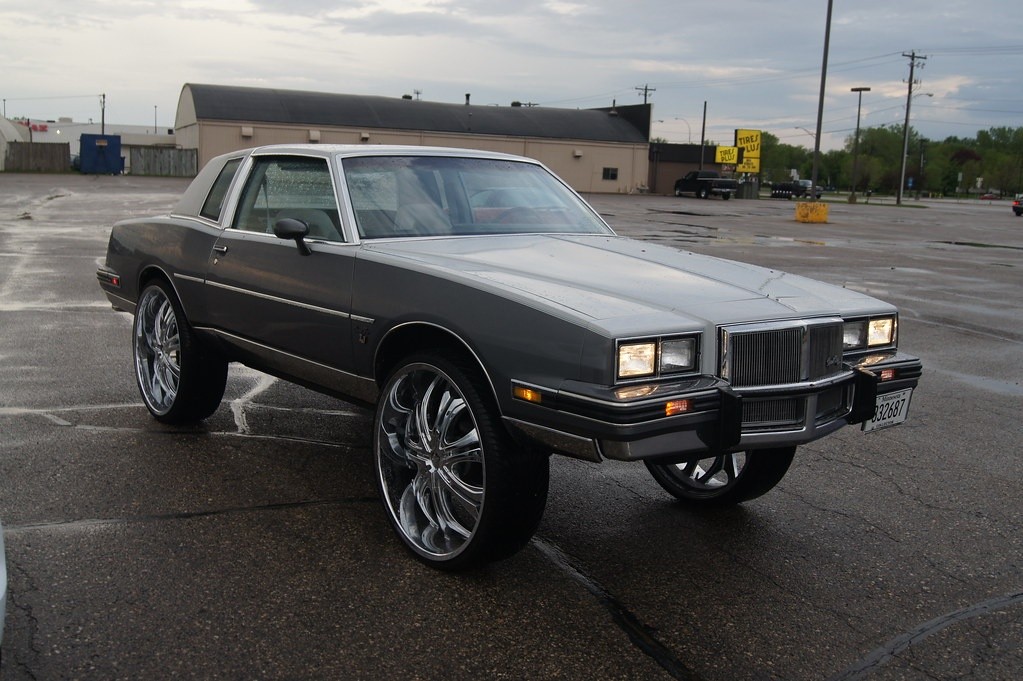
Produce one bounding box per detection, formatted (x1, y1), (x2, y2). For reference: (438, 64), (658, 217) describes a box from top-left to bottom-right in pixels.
(674, 170), (737, 200)
(778, 179), (823, 200)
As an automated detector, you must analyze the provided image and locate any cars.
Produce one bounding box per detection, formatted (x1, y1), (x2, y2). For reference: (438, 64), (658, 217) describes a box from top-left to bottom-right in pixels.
(980, 194), (1000, 200)
(1013, 197), (1023, 217)
(95, 143), (921, 574)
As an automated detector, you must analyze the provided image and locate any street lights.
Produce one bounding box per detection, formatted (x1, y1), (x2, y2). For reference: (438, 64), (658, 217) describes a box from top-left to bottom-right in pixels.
(851, 88), (870, 194)
(899, 93), (934, 204)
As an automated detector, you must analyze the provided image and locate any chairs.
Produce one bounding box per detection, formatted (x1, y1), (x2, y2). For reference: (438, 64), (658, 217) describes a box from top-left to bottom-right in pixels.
(394, 204), (453, 230)
(277, 209), (343, 241)
